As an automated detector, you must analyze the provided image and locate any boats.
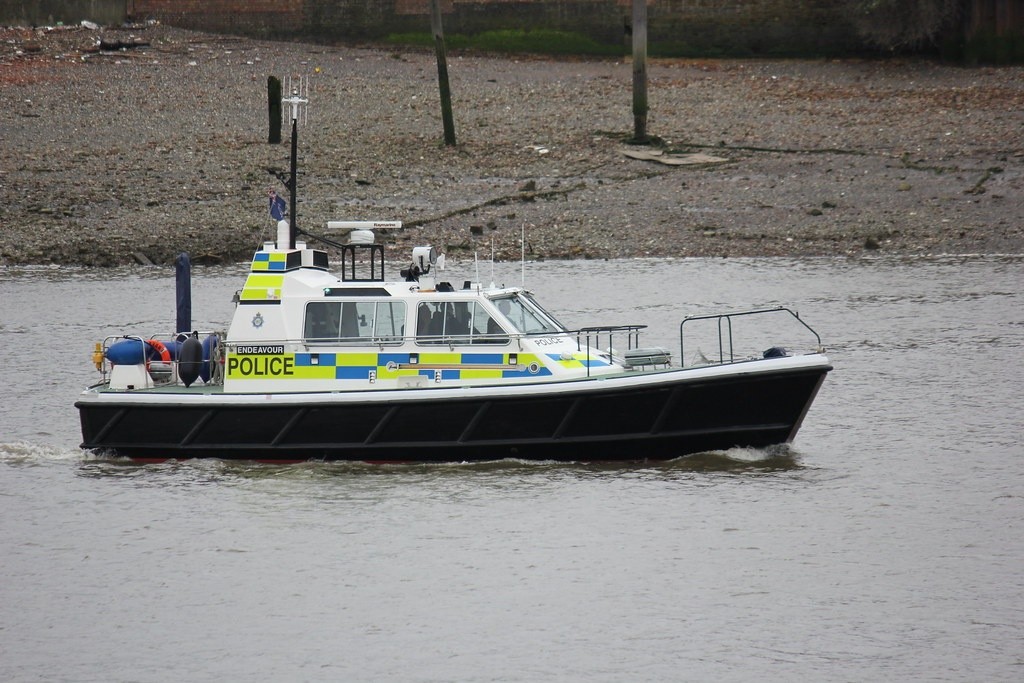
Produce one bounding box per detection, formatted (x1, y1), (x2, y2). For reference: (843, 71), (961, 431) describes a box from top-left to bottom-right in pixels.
(72, 75), (835, 463)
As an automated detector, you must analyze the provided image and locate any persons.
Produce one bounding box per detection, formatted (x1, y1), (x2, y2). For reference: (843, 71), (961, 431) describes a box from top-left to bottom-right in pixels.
(428, 301), (519, 343)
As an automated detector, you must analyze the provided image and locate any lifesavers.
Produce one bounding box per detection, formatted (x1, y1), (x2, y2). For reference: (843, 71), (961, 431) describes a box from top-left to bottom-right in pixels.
(144, 338), (172, 372)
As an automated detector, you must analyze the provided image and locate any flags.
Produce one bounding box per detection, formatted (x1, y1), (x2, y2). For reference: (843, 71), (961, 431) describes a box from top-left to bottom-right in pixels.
(270, 194), (287, 221)
